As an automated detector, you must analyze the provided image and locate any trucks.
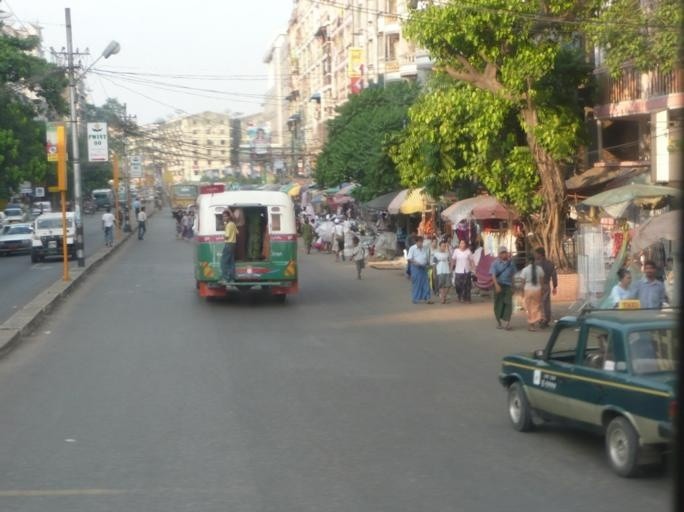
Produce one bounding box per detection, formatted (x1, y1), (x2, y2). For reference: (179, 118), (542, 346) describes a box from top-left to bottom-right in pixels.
(166, 182), (225, 217)
(185, 190), (299, 300)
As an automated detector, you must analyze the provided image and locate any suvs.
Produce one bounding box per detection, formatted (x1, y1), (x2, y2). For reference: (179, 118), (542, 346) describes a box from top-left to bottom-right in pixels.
(31, 212), (76, 261)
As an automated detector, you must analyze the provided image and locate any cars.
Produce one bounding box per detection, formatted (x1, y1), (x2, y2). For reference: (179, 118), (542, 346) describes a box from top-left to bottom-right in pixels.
(497, 300), (681, 478)
(6, 186), (52, 217)
(0, 223), (32, 256)
(89, 179), (164, 222)
(0, 208), (27, 227)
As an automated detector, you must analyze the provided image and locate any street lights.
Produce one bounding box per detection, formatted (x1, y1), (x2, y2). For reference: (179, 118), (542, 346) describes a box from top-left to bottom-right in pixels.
(68, 39), (125, 266)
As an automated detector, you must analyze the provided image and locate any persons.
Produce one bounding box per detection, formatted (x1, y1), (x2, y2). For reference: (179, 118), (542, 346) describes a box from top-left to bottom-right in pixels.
(225, 207), (247, 260)
(172, 209), (196, 239)
(217, 210), (239, 283)
(297, 205), (667, 331)
(101, 208), (116, 247)
(133, 198), (142, 218)
(137, 207), (147, 240)
